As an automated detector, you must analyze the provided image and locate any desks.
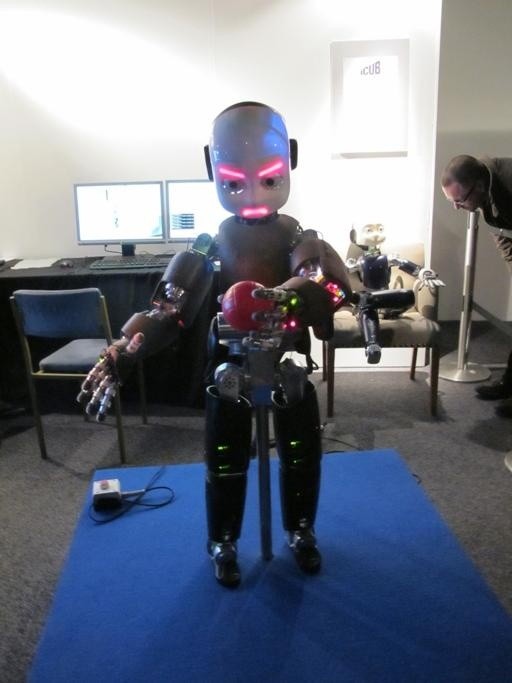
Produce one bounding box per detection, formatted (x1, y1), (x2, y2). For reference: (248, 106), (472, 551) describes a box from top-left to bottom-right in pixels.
(1, 256), (220, 405)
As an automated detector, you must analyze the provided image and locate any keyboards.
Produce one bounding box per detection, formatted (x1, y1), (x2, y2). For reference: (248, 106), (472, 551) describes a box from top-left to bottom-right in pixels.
(90, 257), (171, 269)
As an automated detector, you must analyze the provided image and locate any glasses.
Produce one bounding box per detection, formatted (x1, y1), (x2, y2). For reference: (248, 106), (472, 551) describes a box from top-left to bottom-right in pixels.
(456, 176), (479, 206)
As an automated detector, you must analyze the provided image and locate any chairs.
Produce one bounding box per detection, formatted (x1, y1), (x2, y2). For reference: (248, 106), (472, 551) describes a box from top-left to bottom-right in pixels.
(322, 243), (442, 418)
(12, 287), (148, 464)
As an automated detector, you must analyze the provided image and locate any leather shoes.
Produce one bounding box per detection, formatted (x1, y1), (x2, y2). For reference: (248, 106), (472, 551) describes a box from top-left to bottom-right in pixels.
(475, 381), (512, 417)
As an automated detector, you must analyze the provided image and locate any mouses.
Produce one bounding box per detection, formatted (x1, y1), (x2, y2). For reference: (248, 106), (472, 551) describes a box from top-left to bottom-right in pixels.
(61, 259), (73, 267)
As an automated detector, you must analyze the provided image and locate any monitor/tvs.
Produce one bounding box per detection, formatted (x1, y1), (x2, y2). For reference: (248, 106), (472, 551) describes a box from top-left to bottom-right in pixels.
(166, 178), (235, 245)
(73, 180), (166, 258)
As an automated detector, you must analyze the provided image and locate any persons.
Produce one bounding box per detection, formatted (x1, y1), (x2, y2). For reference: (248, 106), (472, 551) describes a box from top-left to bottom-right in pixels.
(73, 99), (356, 588)
(338, 207), (451, 366)
(439, 152), (512, 417)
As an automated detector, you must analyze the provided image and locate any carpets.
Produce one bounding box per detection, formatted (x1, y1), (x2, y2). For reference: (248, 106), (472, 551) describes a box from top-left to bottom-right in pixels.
(29, 449), (511, 681)
